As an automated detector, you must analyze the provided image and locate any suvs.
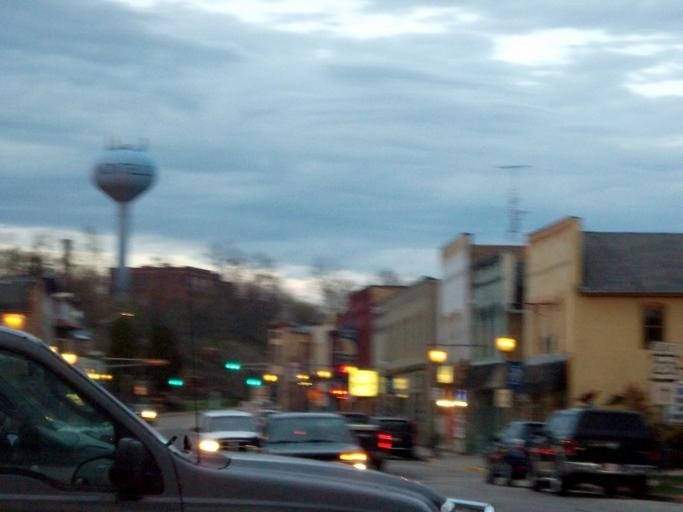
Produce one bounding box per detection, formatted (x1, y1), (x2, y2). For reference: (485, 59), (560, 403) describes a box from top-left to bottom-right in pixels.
(527, 406), (658, 493)
(0, 328), (494, 512)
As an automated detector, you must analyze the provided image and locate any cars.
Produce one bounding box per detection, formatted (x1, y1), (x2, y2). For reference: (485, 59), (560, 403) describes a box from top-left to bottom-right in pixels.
(260, 411), (376, 471)
(486, 422), (545, 485)
(341, 410), (413, 468)
(181, 410), (261, 451)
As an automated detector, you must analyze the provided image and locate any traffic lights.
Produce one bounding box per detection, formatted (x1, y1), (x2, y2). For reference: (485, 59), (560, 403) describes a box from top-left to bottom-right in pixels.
(247, 379), (262, 387)
(168, 379), (184, 387)
(224, 361), (241, 371)
